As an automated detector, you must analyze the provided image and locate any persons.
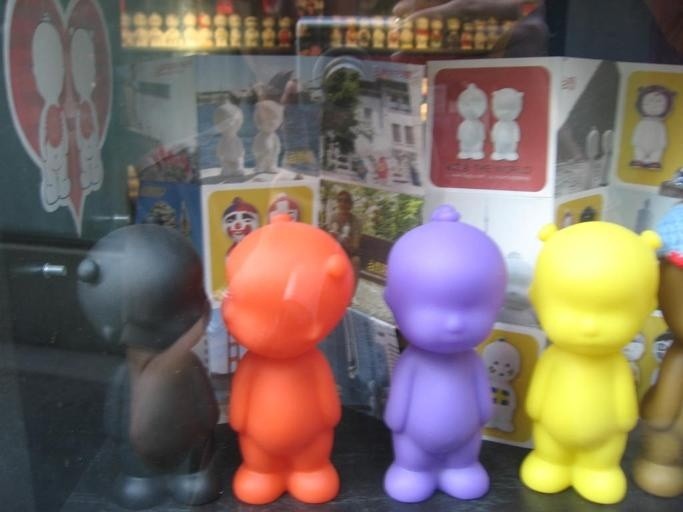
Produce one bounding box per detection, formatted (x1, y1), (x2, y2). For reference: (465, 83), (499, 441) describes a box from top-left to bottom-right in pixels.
(390, 1), (550, 62)
(328, 190), (363, 270)
